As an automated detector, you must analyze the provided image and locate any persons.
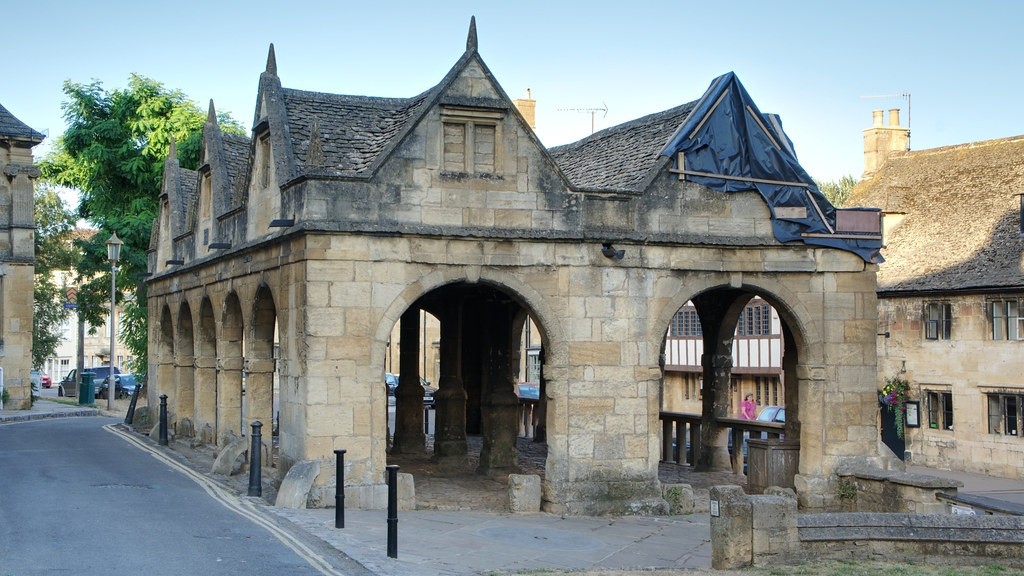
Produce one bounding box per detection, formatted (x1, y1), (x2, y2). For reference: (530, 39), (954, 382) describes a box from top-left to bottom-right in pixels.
(740, 394), (758, 420)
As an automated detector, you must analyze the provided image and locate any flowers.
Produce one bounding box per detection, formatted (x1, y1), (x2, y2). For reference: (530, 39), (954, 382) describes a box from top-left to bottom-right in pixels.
(878, 377), (909, 439)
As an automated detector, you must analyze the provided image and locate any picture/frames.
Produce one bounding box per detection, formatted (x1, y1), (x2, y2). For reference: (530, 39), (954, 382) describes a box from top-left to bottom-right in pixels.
(905, 401), (920, 428)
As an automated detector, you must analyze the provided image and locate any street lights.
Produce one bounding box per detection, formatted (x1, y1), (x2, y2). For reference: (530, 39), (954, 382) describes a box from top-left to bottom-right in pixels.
(106, 230), (125, 409)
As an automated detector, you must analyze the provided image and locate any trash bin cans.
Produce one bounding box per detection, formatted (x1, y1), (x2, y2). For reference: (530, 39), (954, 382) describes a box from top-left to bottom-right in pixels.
(78, 372), (97, 406)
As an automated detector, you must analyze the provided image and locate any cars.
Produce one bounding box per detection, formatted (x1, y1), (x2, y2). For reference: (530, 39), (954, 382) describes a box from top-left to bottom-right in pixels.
(395, 375), (438, 410)
(386, 373), (397, 396)
(30, 368), (51, 389)
(58, 366), (145, 399)
(669, 406), (785, 474)
